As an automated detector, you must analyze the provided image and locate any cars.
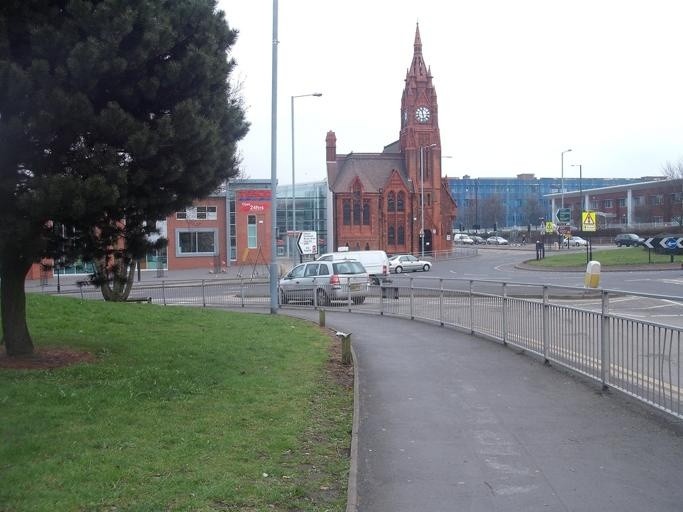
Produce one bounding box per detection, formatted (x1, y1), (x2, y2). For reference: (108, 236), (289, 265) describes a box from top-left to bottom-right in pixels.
(454, 234), (508, 245)
(388, 255), (432, 274)
(614, 233), (645, 247)
(563, 236), (589, 247)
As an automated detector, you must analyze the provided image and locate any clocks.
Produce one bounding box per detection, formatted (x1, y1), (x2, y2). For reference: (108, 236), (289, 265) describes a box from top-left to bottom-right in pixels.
(414, 105), (431, 124)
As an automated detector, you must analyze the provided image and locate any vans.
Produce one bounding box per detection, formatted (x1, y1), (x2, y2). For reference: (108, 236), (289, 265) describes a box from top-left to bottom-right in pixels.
(279, 259), (370, 306)
(317, 250), (390, 285)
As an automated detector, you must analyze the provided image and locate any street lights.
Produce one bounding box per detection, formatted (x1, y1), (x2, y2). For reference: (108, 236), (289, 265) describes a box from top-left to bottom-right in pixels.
(420, 143), (437, 257)
(290, 92), (323, 264)
(561, 149), (573, 236)
(571, 164), (582, 236)
(466, 186), (478, 233)
